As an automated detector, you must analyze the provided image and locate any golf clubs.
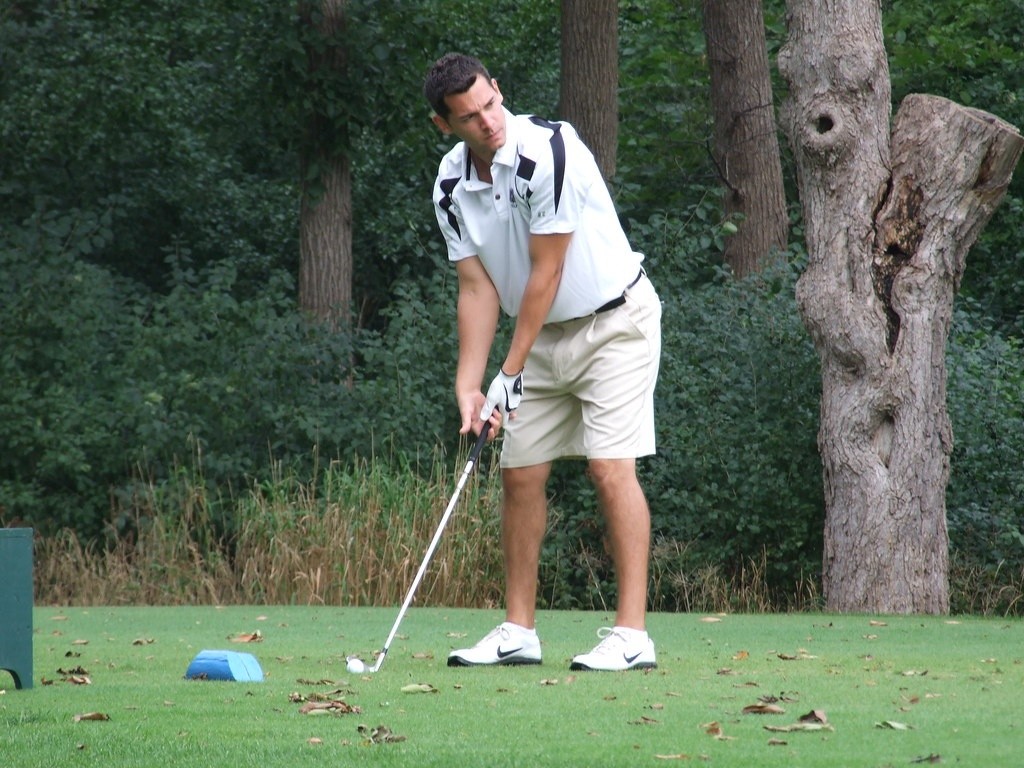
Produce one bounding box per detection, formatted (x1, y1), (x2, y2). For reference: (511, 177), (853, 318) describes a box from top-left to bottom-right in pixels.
(345, 417), (495, 674)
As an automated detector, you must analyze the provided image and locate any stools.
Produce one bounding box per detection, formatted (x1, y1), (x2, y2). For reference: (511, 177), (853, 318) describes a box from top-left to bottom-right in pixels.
(0, 527), (34, 691)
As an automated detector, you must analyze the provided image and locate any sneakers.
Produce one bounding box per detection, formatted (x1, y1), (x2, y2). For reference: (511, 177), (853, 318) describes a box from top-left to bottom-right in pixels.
(569, 626), (657, 671)
(447, 622), (542, 666)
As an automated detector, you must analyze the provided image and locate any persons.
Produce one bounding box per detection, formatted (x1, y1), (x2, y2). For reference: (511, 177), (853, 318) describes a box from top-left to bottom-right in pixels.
(425, 53), (663, 672)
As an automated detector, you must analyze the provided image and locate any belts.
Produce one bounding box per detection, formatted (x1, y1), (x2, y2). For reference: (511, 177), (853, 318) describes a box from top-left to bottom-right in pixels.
(564, 269), (642, 321)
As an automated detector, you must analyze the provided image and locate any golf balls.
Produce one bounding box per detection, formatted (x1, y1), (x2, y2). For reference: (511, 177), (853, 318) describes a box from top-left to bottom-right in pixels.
(347, 659), (364, 675)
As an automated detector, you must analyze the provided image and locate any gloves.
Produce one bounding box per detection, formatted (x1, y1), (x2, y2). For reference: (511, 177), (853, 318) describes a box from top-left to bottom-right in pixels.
(479, 366), (525, 425)
